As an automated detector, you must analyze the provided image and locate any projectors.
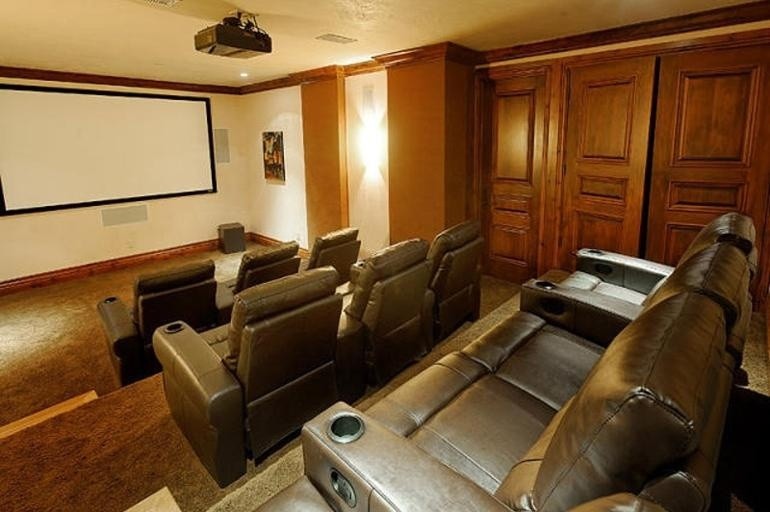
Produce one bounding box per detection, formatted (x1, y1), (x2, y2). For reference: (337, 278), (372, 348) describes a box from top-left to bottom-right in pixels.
(193, 23), (273, 60)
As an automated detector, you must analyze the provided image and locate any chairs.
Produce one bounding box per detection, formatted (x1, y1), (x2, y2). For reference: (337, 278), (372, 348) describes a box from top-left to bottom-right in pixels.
(98, 213), (764, 510)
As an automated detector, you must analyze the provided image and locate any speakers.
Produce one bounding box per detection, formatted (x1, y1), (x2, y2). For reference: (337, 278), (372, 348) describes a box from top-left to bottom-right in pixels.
(218, 222), (246, 254)
